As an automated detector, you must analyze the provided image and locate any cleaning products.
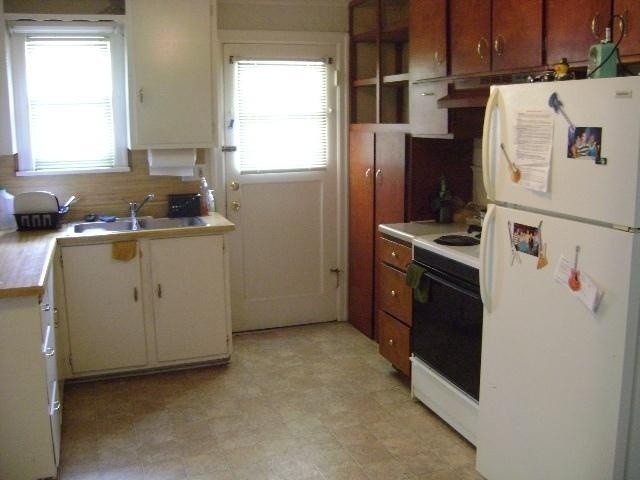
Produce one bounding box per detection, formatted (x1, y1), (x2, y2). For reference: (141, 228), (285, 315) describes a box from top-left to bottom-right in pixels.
(199, 176), (207, 214)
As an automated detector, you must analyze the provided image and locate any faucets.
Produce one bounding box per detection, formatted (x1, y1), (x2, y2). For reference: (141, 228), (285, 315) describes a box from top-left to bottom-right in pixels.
(129, 193), (155, 217)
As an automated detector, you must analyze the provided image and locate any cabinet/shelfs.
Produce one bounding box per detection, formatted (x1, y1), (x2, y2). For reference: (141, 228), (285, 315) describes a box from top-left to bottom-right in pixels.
(448, 0), (543, 78)
(0, 264), (62, 479)
(375, 234), (411, 379)
(348, 0), (408, 342)
(408, 0), (449, 135)
(61, 234), (230, 375)
(543, 1), (639, 71)
(130, 0), (213, 145)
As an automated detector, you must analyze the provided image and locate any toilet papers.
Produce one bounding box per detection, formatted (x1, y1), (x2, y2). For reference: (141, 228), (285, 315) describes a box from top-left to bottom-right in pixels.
(147, 149), (198, 177)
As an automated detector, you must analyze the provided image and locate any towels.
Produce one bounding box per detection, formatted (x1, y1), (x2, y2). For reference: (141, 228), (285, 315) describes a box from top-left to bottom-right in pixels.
(112, 239), (136, 262)
(406, 263), (431, 303)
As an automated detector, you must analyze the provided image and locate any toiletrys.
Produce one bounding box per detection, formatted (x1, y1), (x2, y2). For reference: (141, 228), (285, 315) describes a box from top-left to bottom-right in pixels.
(207, 189), (215, 212)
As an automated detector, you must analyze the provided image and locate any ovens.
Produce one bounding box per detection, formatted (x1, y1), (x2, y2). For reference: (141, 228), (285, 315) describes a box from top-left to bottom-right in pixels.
(409, 259), (484, 448)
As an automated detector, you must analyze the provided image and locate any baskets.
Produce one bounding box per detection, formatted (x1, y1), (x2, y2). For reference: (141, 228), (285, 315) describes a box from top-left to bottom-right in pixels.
(11, 205), (71, 232)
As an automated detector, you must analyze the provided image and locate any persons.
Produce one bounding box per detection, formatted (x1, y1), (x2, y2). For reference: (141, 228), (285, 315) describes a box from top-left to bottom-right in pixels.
(570, 132), (599, 159)
(512, 226), (539, 256)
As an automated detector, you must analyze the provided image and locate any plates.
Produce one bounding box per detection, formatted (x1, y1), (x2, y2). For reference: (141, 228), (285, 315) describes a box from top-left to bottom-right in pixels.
(12, 192), (59, 214)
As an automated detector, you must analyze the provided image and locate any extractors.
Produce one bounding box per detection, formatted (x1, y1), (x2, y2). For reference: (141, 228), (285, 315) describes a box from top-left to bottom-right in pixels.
(438, 89), (488, 107)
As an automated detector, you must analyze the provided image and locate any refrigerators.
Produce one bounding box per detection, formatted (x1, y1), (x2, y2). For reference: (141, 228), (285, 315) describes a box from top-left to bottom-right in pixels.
(474, 77), (640, 479)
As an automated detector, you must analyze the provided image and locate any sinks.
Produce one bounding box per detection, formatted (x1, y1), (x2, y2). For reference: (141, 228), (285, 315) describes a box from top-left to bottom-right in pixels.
(138, 216), (210, 231)
(65, 221), (135, 237)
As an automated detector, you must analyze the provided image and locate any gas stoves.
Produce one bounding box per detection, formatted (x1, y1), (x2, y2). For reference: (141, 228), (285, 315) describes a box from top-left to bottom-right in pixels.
(416, 230), (482, 261)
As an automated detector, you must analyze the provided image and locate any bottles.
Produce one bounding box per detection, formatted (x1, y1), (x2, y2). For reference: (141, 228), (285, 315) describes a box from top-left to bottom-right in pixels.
(437, 176), (452, 223)
(200, 176), (208, 216)
(0, 185), (17, 230)
